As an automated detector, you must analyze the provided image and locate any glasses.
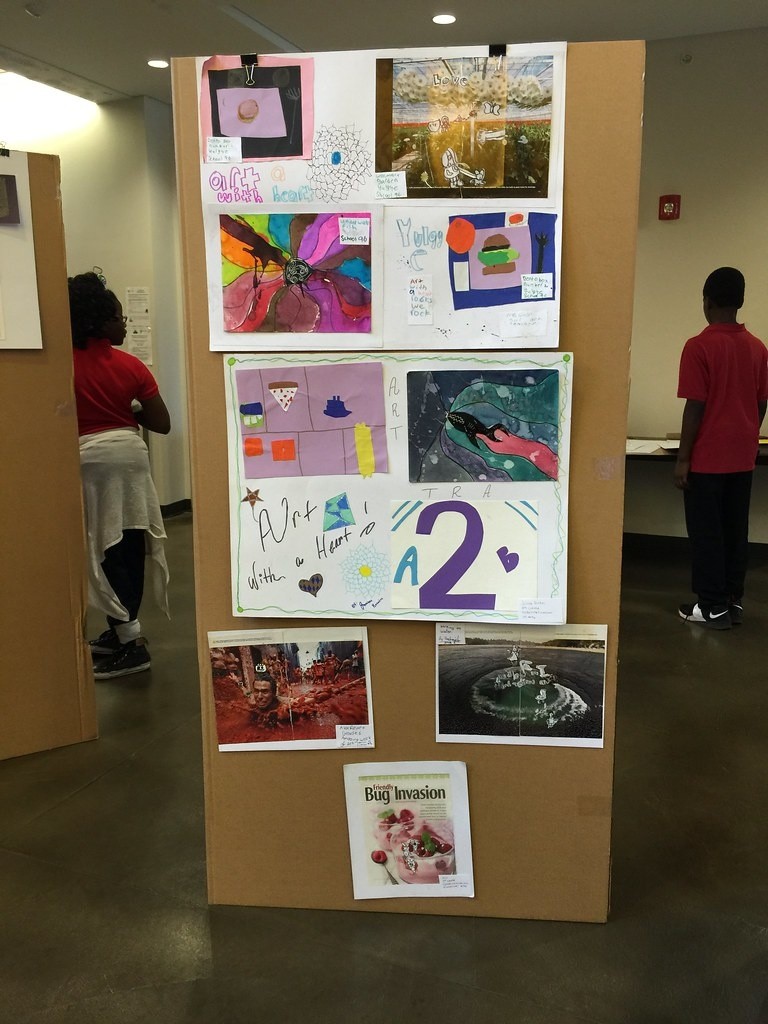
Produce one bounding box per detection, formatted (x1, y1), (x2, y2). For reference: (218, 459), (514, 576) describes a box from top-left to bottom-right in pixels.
(114, 315), (127, 324)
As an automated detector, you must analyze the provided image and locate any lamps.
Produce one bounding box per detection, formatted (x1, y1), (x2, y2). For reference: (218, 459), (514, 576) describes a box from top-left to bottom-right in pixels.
(25, 2), (50, 17)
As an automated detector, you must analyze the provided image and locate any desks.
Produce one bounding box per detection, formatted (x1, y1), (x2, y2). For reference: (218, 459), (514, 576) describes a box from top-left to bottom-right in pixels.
(626, 438), (768, 467)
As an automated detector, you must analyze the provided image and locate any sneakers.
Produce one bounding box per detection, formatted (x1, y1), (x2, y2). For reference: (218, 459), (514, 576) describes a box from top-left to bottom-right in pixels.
(88, 639), (115, 654)
(728, 600), (744, 624)
(678, 602), (732, 629)
(93, 637), (151, 679)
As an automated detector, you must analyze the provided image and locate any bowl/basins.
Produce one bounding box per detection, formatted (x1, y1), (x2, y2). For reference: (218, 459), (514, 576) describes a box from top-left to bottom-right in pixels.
(390, 823), (456, 885)
(372, 813), (419, 852)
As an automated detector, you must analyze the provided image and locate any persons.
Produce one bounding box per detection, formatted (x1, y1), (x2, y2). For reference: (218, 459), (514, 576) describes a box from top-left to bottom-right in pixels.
(67, 271), (172, 679)
(674, 267), (768, 629)
(212, 647), (362, 711)
